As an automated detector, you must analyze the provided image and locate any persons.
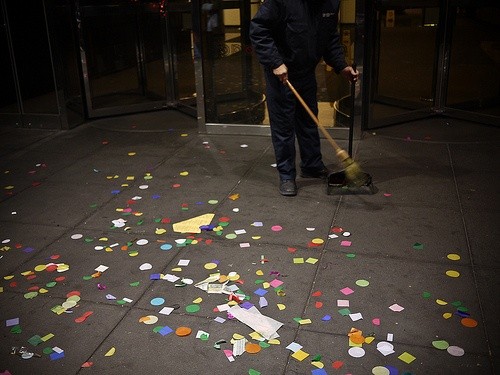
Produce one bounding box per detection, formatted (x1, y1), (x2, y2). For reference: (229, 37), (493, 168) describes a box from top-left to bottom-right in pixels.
(249, 0), (360, 196)
(202, 0), (219, 59)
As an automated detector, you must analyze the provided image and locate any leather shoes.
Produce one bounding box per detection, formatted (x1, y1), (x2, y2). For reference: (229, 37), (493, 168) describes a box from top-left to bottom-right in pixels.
(300, 164), (332, 177)
(278, 177), (297, 195)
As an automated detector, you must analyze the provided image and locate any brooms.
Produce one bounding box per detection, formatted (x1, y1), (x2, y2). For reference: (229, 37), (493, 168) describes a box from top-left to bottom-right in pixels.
(287, 80), (373, 188)
